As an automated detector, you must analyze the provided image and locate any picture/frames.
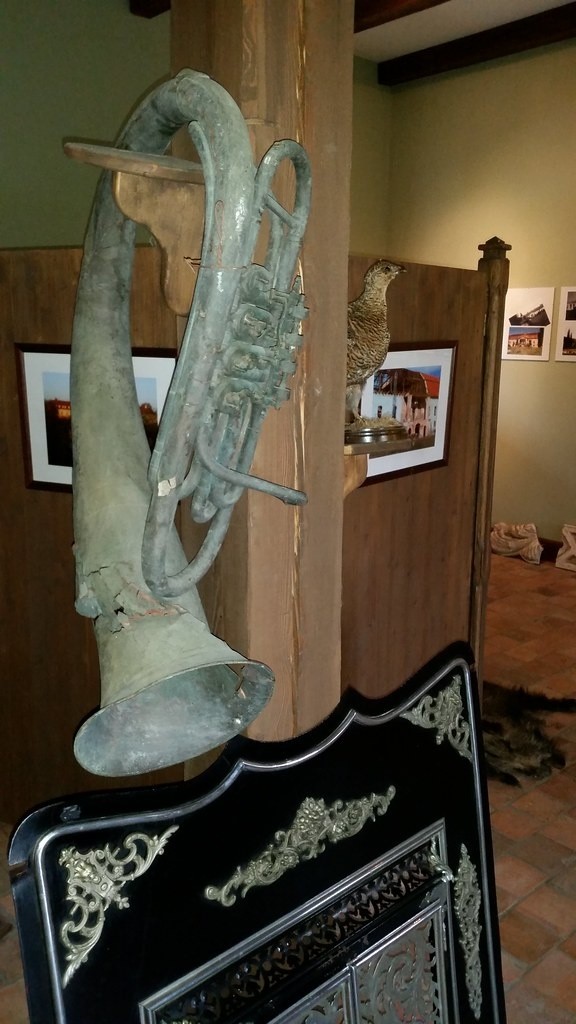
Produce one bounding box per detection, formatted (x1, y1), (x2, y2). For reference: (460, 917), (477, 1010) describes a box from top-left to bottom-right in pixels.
(14, 342), (178, 493)
(345, 340), (460, 488)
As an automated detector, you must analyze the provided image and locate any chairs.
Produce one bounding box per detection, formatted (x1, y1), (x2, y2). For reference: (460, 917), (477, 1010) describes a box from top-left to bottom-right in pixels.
(6, 640), (507, 1024)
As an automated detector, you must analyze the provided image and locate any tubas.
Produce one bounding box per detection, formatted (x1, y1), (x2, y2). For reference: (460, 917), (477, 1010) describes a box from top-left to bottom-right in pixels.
(71, 68), (309, 781)
